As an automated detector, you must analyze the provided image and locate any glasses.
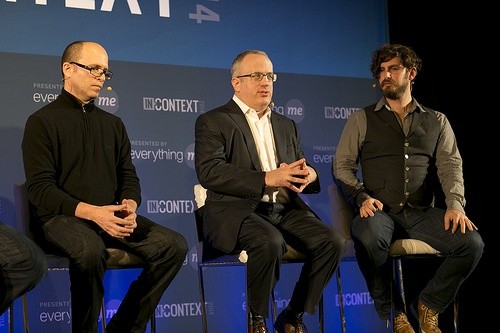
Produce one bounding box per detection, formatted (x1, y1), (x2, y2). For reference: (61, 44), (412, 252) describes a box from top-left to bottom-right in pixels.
(70, 61), (113, 81)
(237, 72), (277, 82)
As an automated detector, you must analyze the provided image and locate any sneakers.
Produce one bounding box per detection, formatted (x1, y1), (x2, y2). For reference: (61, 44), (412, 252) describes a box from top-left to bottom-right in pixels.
(416, 297), (442, 333)
(386, 312), (415, 333)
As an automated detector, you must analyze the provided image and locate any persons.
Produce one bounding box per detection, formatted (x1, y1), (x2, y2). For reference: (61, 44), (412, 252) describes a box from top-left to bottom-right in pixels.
(194, 50), (346, 333)
(331, 44), (485, 333)
(0, 220), (49, 316)
(20, 40), (190, 333)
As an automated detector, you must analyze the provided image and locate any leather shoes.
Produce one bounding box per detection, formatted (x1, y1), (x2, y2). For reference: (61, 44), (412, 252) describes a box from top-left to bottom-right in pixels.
(273, 309), (307, 333)
(249, 310), (267, 333)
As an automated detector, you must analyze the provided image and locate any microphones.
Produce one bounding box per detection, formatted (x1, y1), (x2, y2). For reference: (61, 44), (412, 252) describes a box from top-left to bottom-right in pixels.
(103, 86), (112, 91)
(372, 83), (380, 88)
(271, 100), (275, 107)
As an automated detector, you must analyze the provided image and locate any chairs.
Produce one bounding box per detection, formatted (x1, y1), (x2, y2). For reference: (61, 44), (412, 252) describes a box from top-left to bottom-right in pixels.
(193, 183), (458, 333)
(0, 184), (156, 333)
